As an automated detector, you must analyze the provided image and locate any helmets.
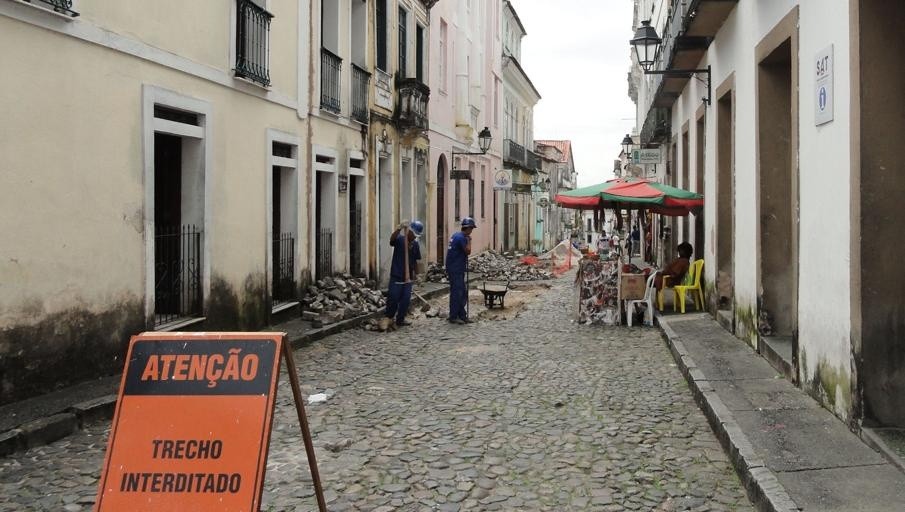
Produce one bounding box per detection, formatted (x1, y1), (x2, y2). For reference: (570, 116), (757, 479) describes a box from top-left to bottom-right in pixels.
(411, 220), (425, 234)
(460, 218), (478, 228)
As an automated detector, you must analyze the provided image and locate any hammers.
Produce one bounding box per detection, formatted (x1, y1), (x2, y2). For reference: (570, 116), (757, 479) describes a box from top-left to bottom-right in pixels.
(395, 227), (417, 285)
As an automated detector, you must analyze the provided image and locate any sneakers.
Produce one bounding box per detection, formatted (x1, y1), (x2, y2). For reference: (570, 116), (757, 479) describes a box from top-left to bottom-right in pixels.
(395, 317), (412, 326)
(450, 315), (473, 325)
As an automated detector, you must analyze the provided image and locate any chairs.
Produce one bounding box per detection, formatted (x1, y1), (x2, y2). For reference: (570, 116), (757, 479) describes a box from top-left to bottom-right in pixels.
(658, 259), (706, 315)
(625, 269), (661, 326)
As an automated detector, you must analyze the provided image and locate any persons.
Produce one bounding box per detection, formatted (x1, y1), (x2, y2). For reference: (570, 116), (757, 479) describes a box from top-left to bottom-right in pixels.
(635, 242), (692, 317)
(596, 225), (640, 255)
(385, 220), (423, 326)
(446, 218), (477, 324)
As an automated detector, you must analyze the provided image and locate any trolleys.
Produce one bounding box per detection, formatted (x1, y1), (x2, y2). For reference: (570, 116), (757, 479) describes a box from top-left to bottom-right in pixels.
(477, 280), (511, 308)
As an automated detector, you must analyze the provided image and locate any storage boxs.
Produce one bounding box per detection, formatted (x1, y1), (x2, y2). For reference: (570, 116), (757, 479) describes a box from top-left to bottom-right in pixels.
(622, 273), (646, 300)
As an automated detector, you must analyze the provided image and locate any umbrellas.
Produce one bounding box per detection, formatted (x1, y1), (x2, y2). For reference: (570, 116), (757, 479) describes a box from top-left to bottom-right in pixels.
(554, 176), (704, 262)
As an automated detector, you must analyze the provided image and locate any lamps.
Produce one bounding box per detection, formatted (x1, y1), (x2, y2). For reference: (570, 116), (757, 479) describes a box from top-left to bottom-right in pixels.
(454, 127), (493, 156)
(630, 18), (712, 107)
(621, 133), (636, 161)
